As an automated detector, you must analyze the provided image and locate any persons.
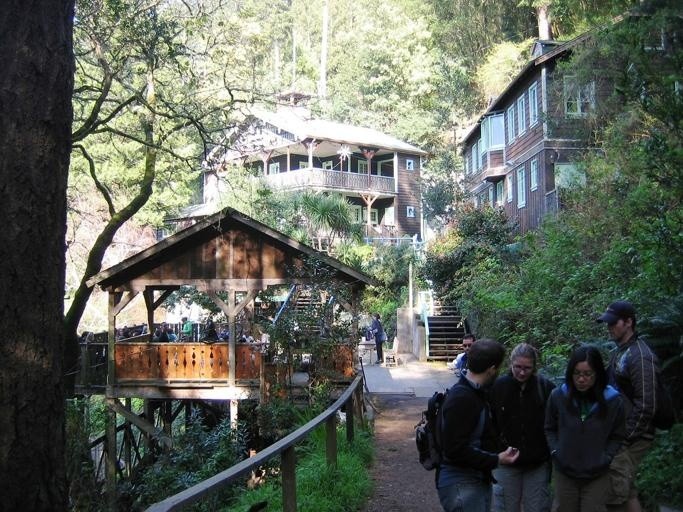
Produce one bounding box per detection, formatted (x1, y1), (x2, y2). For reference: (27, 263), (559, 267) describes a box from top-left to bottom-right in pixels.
(370, 314), (383, 364)
(436, 302), (655, 512)
(82, 317), (270, 344)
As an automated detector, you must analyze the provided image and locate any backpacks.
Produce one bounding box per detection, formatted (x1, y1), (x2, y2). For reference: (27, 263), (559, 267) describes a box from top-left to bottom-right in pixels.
(413, 386), (443, 472)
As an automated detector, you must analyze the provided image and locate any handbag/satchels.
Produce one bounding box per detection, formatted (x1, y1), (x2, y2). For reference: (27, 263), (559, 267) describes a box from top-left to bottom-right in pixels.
(377, 331), (388, 342)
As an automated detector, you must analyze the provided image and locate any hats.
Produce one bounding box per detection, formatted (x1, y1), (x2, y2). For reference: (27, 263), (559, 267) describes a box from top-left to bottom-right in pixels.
(595, 301), (634, 323)
